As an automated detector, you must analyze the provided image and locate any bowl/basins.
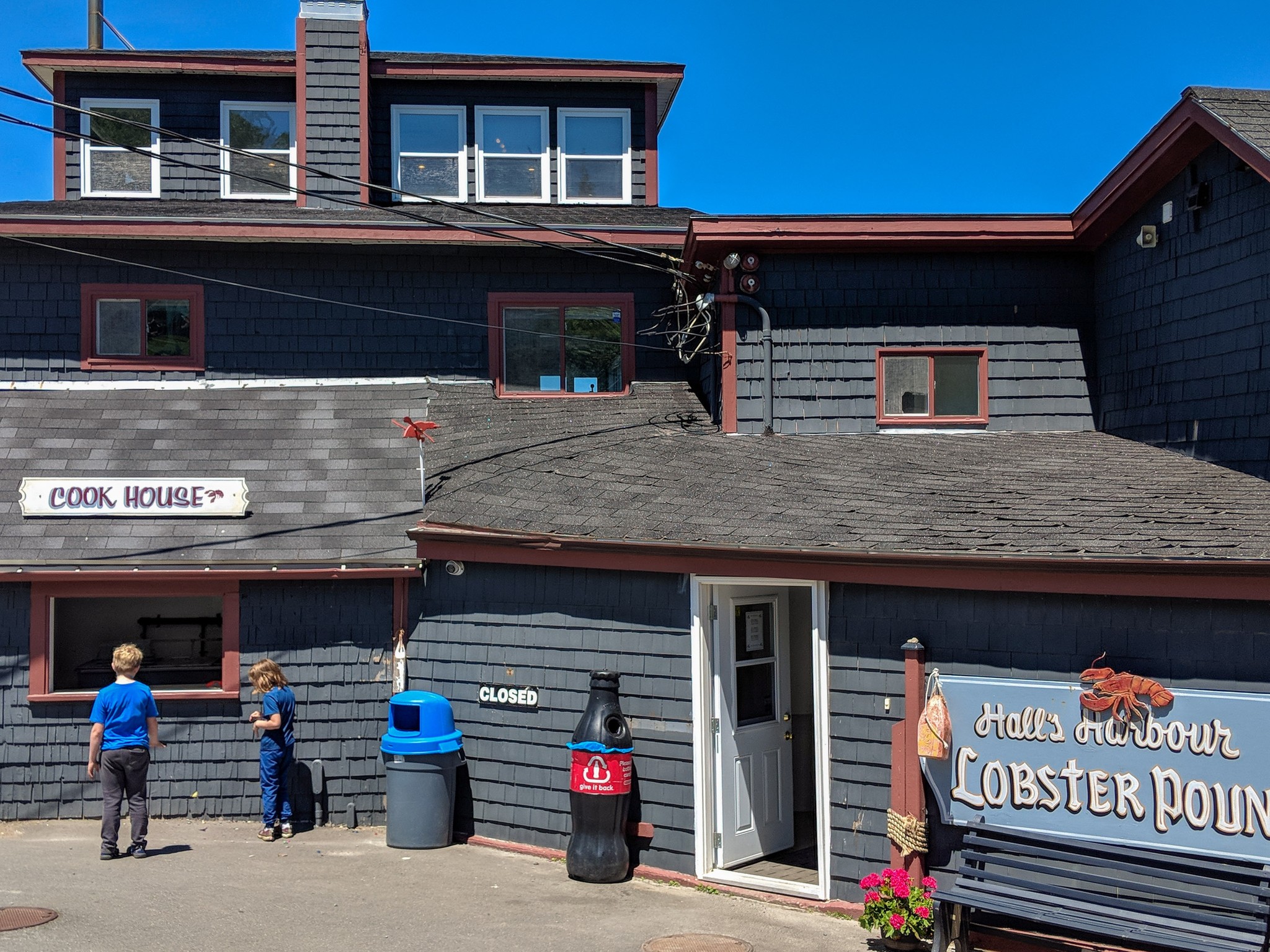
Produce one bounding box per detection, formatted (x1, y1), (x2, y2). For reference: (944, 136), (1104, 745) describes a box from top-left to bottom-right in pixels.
(199, 656), (215, 666)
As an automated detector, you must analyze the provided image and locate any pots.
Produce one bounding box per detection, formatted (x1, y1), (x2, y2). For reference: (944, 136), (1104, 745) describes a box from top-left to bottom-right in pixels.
(171, 652), (195, 665)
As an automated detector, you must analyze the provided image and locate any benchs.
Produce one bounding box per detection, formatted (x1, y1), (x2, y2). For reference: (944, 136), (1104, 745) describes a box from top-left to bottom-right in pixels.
(931, 813), (1270, 952)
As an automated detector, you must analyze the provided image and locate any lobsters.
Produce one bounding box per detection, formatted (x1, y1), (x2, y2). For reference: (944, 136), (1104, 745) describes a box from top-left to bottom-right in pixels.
(1080, 668), (1175, 722)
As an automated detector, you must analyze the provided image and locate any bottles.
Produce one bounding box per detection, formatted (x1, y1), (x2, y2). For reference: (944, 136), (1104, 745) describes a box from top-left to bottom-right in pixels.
(565, 670), (633, 883)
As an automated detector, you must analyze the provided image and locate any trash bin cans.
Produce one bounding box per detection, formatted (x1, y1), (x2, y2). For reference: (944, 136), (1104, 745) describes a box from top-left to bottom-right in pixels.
(380, 691), (468, 849)
(565, 670), (633, 882)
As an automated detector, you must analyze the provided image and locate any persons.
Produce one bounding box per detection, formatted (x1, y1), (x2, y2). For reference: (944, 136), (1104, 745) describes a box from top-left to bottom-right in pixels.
(88, 643), (166, 860)
(248, 658), (295, 841)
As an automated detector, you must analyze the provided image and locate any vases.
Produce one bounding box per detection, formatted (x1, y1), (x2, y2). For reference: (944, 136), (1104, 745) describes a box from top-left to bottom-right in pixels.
(880, 927), (922, 952)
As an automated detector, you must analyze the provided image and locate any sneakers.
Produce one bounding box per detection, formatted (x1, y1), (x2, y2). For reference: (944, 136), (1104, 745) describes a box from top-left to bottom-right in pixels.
(101, 848), (119, 860)
(127, 845), (147, 858)
(257, 829), (275, 840)
(274, 825), (293, 838)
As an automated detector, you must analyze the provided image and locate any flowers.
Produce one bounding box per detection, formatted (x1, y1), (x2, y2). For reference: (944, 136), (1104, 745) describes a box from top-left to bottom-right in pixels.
(858, 867), (936, 940)
